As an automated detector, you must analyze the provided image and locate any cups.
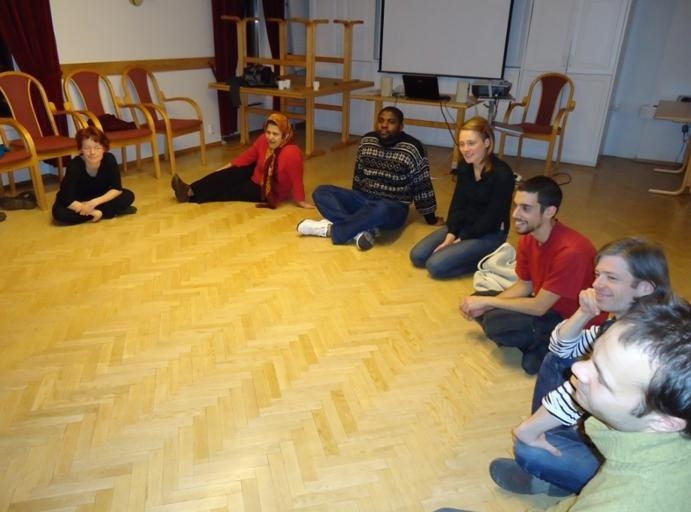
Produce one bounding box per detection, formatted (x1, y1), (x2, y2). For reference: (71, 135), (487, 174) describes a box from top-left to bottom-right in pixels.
(276, 79), (320, 91)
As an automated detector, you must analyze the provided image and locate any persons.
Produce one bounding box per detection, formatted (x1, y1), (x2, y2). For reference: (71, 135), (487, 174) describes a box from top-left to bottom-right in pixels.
(408, 114), (517, 277)
(294, 104), (447, 250)
(51, 126), (135, 225)
(459, 173), (610, 375)
(435, 296), (690, 512)
(490, 235), (674, 494)
(170, 112), (318, 210)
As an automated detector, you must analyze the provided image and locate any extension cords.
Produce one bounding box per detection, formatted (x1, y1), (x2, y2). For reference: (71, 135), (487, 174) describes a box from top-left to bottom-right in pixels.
(512, 171), (522, 183)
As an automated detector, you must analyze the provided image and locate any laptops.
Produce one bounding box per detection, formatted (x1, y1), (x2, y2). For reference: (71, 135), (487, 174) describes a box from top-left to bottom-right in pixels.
(401, 75), (451, 100)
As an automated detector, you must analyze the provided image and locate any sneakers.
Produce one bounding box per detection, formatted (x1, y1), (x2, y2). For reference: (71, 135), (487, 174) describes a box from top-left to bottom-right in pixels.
(490, 458), (573, 497)
(172, 175), (190, 202)
(0, 191), (37, 210)
(297, 218), (334, 238)
(354, 227), (381, 250)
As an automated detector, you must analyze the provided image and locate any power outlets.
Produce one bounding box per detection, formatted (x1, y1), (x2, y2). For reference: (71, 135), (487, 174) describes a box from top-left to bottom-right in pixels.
(680, 123), (690, 133)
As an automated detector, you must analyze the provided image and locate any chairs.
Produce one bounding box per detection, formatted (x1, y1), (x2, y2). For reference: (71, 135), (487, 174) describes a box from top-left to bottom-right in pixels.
(63, 67), (161, 180)
(1, 71), (88, 195)
(490, 73), (576, 176)
(122, 65), (207, 175)
(0, 117), (48, 211)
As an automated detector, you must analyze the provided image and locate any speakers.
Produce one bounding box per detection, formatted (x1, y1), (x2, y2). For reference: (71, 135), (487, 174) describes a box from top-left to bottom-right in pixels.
(381, 77), (392, 97)
(456, 81), (470, 102)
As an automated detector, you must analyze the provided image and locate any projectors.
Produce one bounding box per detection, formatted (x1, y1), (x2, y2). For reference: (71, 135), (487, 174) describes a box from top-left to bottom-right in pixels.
(472, 80), (512, 98)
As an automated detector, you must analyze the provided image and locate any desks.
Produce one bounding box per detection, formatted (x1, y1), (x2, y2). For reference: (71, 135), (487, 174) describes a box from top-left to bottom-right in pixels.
(208, 15), (375, 160)
(647, 100), (691, 196)
(345, 89), (486, 170)
